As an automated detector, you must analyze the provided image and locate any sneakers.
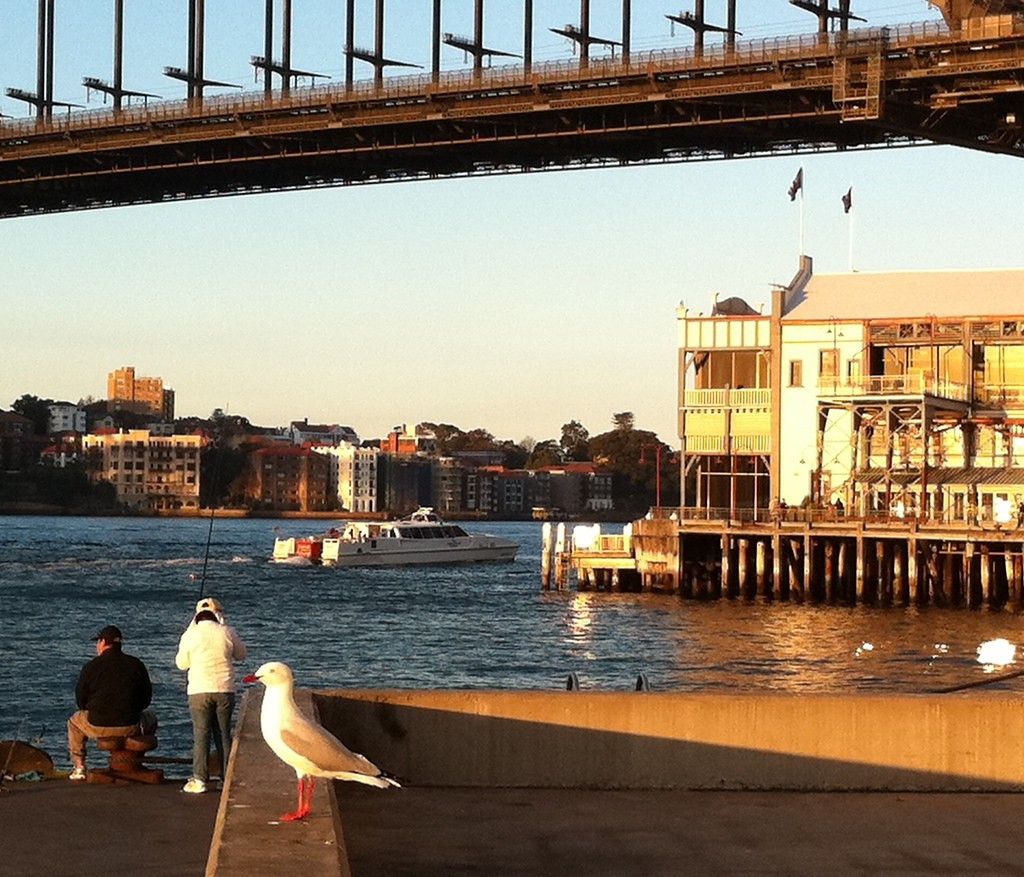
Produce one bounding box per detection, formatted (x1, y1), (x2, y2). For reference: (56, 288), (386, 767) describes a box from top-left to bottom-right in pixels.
(69, 765), (86, 780)
(183, 778), (209, 793)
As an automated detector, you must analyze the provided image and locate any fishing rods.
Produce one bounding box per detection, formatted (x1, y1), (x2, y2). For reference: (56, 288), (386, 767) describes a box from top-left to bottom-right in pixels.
(199, 403), (231, 600)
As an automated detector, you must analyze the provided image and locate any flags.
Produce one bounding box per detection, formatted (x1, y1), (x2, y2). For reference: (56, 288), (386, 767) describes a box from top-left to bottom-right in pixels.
(788, 168), (801, 201)
(842, 187), (851, 213)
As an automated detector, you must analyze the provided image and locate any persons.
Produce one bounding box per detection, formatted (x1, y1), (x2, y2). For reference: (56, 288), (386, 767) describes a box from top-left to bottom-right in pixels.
(67, 625), (153, 779)
(835, 498), (843, 516)
(780, 499), (789, 521)
(1017, 501), (1024, 527)
(176, 599), (246, 792)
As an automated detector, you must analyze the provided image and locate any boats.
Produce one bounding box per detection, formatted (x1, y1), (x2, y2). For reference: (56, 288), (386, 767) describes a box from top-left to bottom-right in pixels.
(265, 520), (347, 568)
(317, 503), (522, 571)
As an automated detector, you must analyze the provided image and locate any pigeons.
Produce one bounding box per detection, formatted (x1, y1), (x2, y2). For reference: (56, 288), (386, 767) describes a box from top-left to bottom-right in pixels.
(243, 662), (413, 821)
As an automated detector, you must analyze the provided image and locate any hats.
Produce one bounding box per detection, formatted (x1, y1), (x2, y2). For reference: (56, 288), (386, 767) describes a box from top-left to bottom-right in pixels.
(90, 626), (122, 643)
(196, 597), (222, 611)
